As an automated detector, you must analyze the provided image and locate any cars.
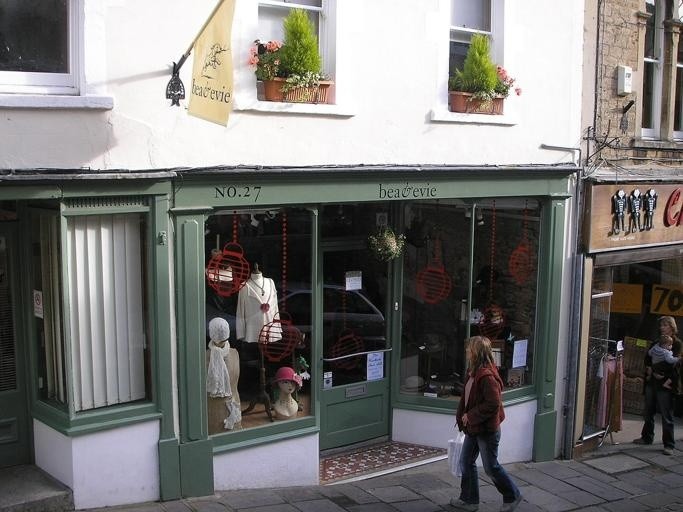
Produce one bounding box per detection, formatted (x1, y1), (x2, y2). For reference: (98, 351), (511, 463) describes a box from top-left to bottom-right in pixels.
(205, 282), (432, 394)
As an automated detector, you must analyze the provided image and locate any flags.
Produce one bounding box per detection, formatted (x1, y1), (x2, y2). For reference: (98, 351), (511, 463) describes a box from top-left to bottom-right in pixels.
(187, 2), (236, 127)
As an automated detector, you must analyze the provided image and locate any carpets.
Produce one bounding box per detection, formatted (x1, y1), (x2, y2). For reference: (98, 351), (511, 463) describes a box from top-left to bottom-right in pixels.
(319, 441), (448, 484)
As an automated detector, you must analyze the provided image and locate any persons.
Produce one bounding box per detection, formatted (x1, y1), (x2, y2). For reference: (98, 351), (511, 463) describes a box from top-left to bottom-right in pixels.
(235, 272), (283, 343)
(271, 367), (303, 420)
(633, 316), (683, 455)
(206, 318), (241, 435)
(646, 335), (682, 388)
(450, 336), (522, 512)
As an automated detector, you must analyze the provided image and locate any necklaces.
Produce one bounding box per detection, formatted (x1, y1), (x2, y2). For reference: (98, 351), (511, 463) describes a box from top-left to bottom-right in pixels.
(251, 277), (265, 297)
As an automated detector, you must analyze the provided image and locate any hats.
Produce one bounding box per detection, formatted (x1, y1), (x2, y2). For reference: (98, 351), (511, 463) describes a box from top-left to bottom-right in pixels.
(270, 367), (303, 390)
(401, 376), (427, 392)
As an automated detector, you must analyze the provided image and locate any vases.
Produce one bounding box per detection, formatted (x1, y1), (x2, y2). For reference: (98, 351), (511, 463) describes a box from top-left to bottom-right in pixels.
(262, 76), (333, 104)
(449, 90), (505, 115)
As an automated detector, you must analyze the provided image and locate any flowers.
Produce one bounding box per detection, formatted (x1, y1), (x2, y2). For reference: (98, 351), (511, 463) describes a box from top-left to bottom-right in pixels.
(449, 33), (522, 95)
(250, 7), (334, 78)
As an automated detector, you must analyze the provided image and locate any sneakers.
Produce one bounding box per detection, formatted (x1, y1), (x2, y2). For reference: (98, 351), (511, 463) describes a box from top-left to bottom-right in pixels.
(500, 493), (523, 512)
(663, 446), (673, 455)
(633, 438), (646, 444)
(450, 497), (479, 512)
